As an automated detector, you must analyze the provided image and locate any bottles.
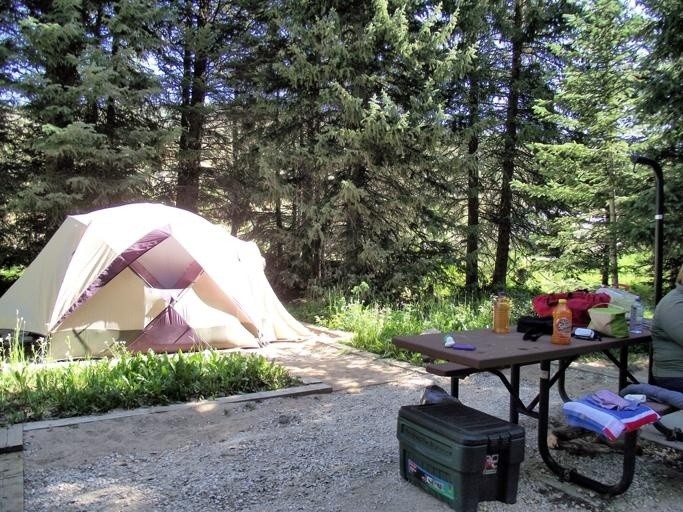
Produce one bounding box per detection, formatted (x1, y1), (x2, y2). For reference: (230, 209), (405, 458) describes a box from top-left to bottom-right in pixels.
(628, 294), (644, 333)
(492, 291), (512, 334)
(550, 298), (572, 343)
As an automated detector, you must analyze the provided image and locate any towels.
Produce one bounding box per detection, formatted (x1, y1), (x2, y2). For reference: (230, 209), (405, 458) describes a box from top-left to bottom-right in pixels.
(588, 388), (638, 411)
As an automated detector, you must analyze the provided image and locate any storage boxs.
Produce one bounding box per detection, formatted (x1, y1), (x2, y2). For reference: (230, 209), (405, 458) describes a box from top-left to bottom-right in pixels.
(396, 397), (527, 511)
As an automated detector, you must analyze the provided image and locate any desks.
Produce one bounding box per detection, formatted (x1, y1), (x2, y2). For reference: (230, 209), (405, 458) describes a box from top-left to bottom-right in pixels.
(390, 325), (650, 493)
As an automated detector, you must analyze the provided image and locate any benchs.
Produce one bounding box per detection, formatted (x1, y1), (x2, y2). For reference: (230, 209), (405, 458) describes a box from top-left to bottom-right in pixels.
(562, 386), (683, 494)
(425, 360), (580, 403)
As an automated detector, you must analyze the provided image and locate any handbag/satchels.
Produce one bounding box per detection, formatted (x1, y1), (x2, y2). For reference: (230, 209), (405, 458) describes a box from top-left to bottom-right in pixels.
(586, 302), (630, 339)
(516, 289), (612, 343)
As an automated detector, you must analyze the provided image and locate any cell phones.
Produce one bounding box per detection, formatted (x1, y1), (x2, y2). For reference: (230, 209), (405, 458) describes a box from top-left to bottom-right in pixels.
(452, 343), (476, 350)
(575, 328), (594, 339)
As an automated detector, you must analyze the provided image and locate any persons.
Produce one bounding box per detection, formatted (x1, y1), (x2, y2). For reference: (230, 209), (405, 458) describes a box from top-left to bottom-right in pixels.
(651, 263), (683, 392)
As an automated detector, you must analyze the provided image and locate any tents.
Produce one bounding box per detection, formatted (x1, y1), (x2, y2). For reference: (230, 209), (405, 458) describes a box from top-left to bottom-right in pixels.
(0, 204), (311, 361)
